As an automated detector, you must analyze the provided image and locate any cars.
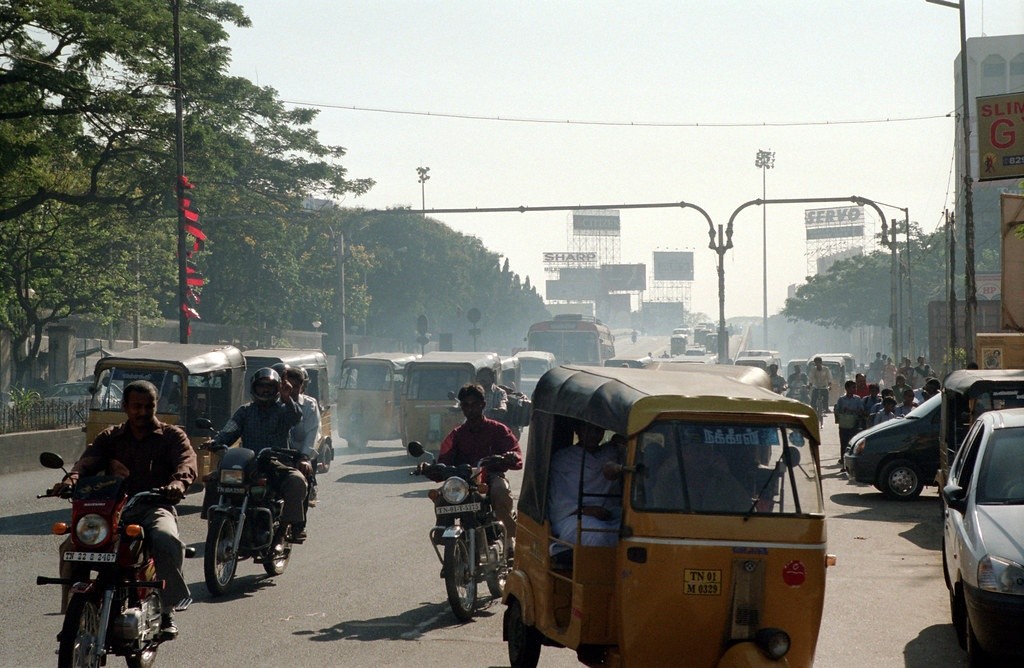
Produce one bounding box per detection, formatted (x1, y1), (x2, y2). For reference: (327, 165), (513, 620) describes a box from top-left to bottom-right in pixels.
(603, 321), (856, 406)
(843, 388), (942, 502)
(0, 375), (123, 424)
(942, 408), (1024, 668)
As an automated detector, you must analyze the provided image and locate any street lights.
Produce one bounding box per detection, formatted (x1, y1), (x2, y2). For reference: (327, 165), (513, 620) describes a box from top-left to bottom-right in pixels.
(753, 149), (777, 349)
(311, 320), (322, 331)
(415, 166), (431, 257)
(22, 281), (36, 389)
(341, 241), (380, 363)
(362, 245), (407, 336)
(857, 196), (912, 359)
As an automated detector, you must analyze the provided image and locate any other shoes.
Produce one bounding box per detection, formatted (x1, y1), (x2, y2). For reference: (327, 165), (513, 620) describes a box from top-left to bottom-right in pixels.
(291, 530), (307, 541)
(272, 535), (285, 554)
(160, 622), (178, 638)
(506, 547), (514, 567)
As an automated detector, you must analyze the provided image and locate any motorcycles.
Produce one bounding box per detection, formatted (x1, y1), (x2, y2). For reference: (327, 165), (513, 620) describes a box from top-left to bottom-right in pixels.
(334, 350), (556, 460)
(501, 365), (839, 667)
(408, 441), (519, 622)
(194, 416), (316, 598)
(37, 451), (198, 667)
(939, 368), (1024, 518)
(210, 349), (335, 473)
(82, 342), (247, 492)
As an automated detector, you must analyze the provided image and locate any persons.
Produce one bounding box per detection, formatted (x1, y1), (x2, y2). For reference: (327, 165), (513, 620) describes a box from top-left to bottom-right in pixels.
(546, 419), (756, 547)
(631, 328), (638, 341)
(769, 364), (786, 395)
(809, 356), (832, 414)
(647, 350), (679, 359)
(786, 365), (810, 405)
(835, 352), (990, 465)
(449, 367), (508, 421)
(144, 369), (181, 413)
(276, 368), (322, 541)
(622, 363), (629, 368)
(49, 380), (197, 643)
(200, 368), (308, 557)
(414, 383), (523, 568)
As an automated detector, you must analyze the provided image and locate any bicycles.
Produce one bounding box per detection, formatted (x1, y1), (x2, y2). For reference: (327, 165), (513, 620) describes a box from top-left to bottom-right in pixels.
(810, 385), (831, 429)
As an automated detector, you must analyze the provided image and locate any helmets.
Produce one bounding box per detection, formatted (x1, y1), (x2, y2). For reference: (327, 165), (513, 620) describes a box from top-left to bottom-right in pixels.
(271, 363), (290, 378)
(250, 368), (280, 403)
(293, 366), (309, 381)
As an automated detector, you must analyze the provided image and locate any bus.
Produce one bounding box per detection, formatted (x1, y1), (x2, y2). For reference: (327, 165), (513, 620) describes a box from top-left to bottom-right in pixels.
(523, 313), (616, 367)
